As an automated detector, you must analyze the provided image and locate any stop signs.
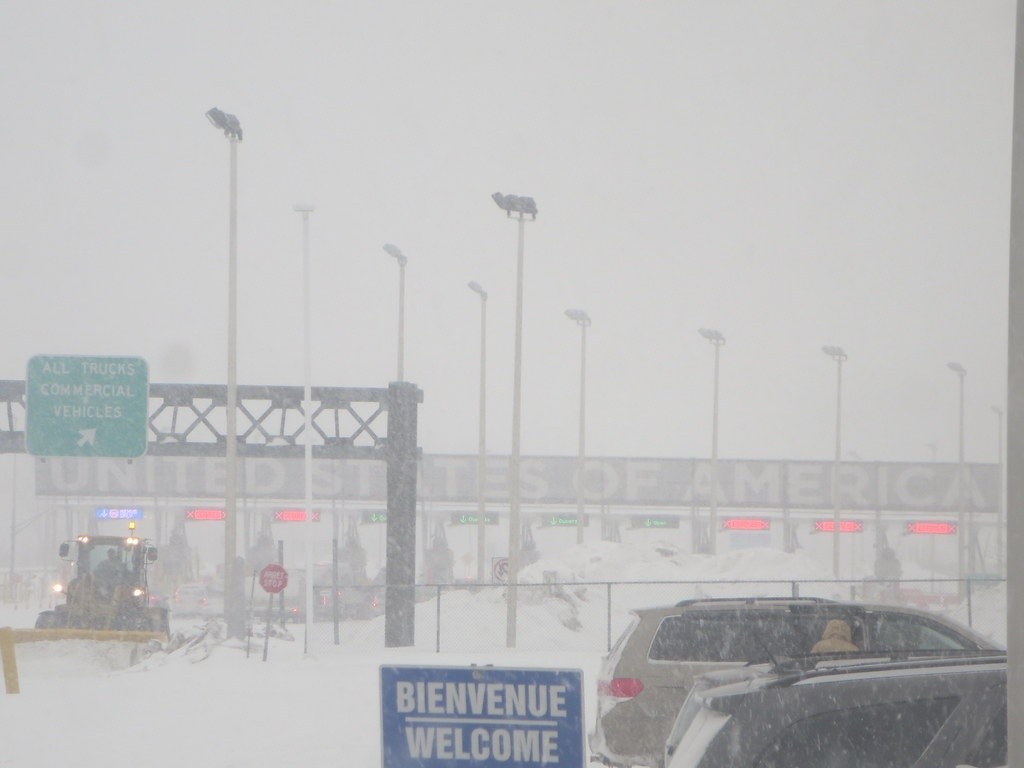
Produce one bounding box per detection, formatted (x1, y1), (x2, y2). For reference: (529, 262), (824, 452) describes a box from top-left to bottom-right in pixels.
(259, 565), (288, 595)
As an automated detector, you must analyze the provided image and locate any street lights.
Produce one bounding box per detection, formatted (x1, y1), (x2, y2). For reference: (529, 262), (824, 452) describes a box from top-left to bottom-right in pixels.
(700, 329), (723, 549)
(948, 361), (968, 592)
(824, 347), (845, 576)
(207, 108), (244, 638)
(295, 204), (315, 654)
(567, 307), (587, 541)
(382, 243), (408, 383)
(493, 193), (539, 645)
(469, 283), (488, 577)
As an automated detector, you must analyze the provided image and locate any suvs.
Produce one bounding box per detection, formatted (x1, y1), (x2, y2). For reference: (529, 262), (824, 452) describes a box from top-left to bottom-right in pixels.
(589, 597), (1008, 768)
(664, 650), (1007, 768)
(669, 658), (1007, 768)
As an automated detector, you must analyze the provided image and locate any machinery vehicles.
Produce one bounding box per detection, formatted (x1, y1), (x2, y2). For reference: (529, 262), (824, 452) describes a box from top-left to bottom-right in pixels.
(33, 522), (171, 640)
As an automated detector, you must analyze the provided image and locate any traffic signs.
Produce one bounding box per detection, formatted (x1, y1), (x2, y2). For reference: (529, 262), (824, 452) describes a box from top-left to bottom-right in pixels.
(24, 358), (149, 459)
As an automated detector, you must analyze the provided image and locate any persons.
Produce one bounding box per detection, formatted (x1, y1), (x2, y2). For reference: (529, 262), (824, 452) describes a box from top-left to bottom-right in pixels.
(810, 618), (859, 655)
(93, 549), (123, 580)
